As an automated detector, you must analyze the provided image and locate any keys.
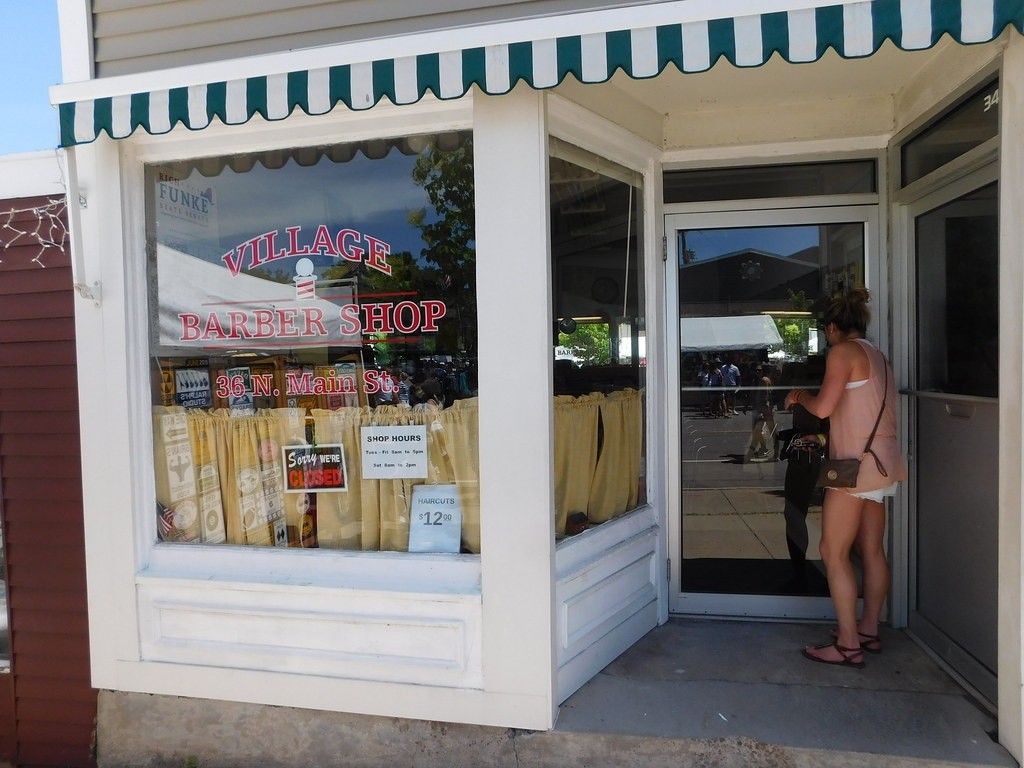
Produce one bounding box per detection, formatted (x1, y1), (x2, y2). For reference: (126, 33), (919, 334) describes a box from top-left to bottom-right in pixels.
(785, 434), (819, 463)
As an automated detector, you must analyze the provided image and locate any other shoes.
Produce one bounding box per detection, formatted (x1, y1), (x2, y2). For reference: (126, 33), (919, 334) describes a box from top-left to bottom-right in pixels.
(768, 455), (780, 462)
(753, 448), (771, 458)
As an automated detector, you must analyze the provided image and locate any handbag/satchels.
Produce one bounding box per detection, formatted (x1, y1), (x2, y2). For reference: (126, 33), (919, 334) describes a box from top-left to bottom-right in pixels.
(814, 458), (861, 489)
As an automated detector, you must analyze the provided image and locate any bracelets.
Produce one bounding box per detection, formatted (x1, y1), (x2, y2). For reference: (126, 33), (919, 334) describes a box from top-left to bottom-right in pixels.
(792, 388), (809, 403)
(817, 434), (827, 447)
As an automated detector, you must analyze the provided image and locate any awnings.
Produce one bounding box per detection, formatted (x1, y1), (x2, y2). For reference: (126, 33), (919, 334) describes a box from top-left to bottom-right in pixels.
(55, 1), (1024, 156)
(680, 314), (784, 352)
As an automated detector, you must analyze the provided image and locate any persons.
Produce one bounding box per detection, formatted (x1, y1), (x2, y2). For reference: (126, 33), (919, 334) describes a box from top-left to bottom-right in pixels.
(368, 354), (470, 408)
(783, 285), (907, 668)
(746, 377), (780, 462)
(740, 361), (775, 458)
(779, 353), (831, 564)
(697, 354), (742, 418)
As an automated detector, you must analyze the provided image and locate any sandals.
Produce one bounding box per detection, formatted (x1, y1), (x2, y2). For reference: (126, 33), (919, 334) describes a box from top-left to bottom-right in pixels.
(830, 627), (882, 654)
(802, 639), (865, 668)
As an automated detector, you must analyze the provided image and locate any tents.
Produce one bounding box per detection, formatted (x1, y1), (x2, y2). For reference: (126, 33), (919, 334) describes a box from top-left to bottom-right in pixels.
(156, 239), (363, 356)
